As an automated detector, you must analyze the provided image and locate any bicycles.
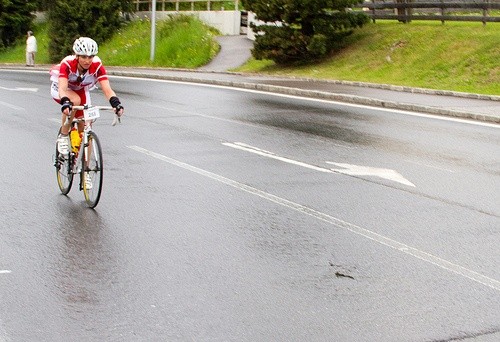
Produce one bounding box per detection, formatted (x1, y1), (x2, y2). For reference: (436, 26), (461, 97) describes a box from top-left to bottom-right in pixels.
(52, 104), (119, 209)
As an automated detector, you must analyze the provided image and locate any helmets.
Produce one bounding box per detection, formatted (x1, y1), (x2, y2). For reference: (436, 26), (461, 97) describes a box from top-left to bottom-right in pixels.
(73, 37), (99, 56)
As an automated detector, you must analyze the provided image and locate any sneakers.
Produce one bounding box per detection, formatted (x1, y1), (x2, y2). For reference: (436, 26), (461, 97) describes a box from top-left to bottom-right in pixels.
(82, 171), (93, 190)
(57, 134), (70, 155)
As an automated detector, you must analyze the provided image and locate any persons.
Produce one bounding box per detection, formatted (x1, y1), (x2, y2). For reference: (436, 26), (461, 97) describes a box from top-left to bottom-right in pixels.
(26, 31), (37, 67)
(50, 37), (124, 190)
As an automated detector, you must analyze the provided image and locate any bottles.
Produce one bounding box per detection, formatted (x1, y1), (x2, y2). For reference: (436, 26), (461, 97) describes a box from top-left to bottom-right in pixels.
(71, 127), (79, 152)
(74, 131), (84, 153)
